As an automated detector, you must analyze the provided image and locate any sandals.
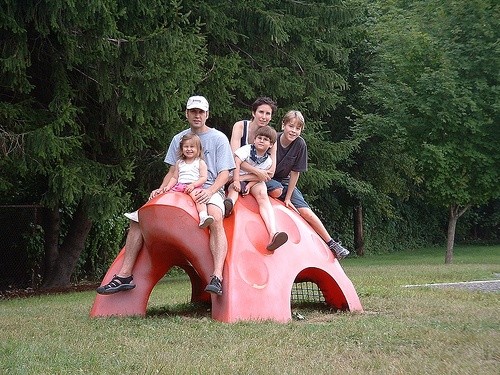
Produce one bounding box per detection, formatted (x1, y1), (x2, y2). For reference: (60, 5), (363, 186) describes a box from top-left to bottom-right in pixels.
(96, 273), (136, 295)
(203, 274), (223, 296)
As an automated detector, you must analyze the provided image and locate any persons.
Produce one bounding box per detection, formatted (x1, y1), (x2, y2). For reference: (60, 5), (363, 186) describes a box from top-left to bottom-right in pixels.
(124, 133), (214, 229)
(224, 126), (276, 218)
(266, 110), (350, 262)
(97, 96), (237, 296)
(230, 97), (288, 251)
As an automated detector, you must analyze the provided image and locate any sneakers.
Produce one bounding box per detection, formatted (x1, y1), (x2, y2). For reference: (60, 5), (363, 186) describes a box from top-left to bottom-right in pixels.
(330, 241), (350, 260)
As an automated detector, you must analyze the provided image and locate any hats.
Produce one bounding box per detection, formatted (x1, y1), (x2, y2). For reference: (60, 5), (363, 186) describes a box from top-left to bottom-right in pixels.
(186, 96), (209, 111)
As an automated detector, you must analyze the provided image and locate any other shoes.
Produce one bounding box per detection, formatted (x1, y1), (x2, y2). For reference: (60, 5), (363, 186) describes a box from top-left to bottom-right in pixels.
(198, 216), (214, 228)
(124, 210), (138, 222)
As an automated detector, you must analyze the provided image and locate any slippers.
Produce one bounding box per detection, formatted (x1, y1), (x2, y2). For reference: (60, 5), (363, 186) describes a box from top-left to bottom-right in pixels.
(224, 198), (233, 217)
(266, 232), (288, 251)
